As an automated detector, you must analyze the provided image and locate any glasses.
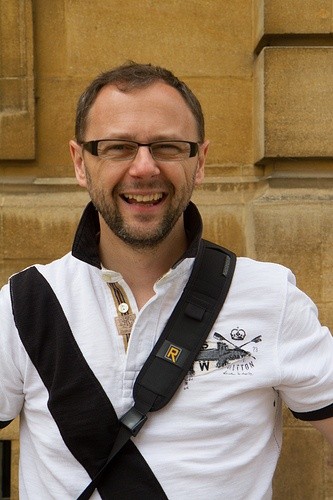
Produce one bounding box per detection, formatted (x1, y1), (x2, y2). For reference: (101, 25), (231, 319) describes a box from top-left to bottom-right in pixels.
(77, 139), (201, 162)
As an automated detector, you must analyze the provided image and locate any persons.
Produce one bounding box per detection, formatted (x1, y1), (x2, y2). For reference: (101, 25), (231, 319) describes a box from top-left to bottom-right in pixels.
(0, 60), (333, 500)
